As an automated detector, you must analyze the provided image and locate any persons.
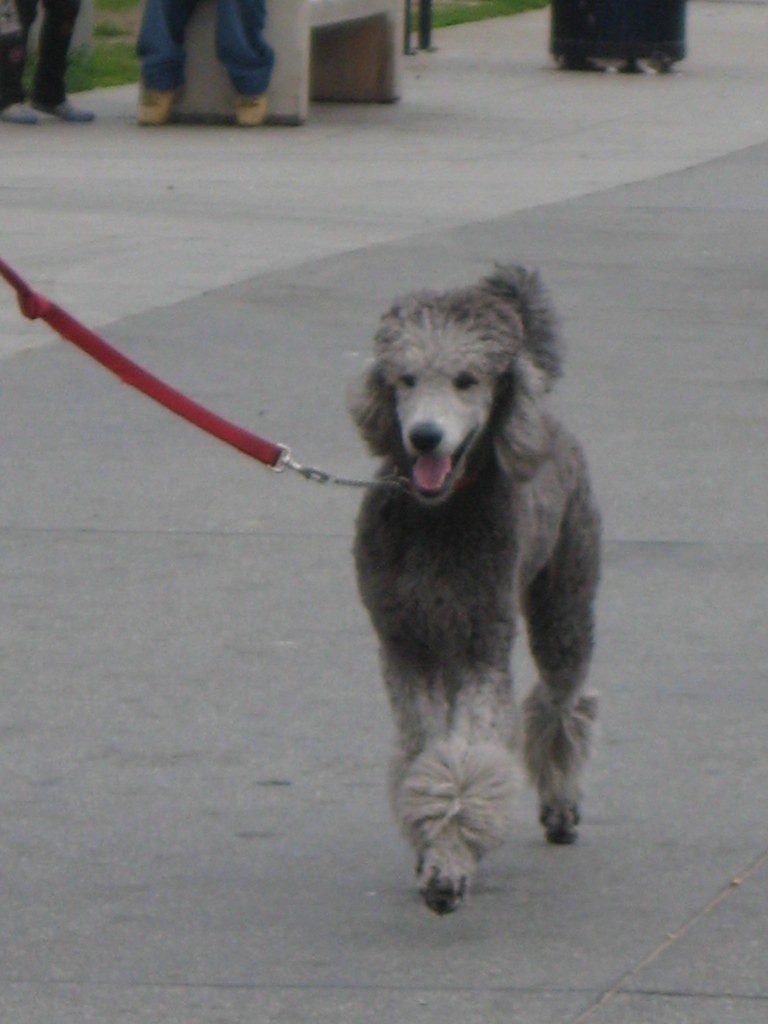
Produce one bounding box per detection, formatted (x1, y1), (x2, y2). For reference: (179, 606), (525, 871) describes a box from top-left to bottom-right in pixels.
(0, 0), (95, 124)
(134, 0), (276, 127)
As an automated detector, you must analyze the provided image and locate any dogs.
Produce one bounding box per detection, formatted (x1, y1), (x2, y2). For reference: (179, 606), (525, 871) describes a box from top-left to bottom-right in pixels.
(346, 265), (607, 917)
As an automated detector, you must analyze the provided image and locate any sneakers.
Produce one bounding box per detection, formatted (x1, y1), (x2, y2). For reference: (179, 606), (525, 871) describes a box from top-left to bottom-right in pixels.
(134, 85), (172, 127)
(232, 94), (271, 127)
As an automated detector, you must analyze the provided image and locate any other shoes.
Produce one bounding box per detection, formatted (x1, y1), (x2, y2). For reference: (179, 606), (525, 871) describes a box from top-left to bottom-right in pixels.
(31, 96), (96, 123)
(3, 99), (43, 126)
